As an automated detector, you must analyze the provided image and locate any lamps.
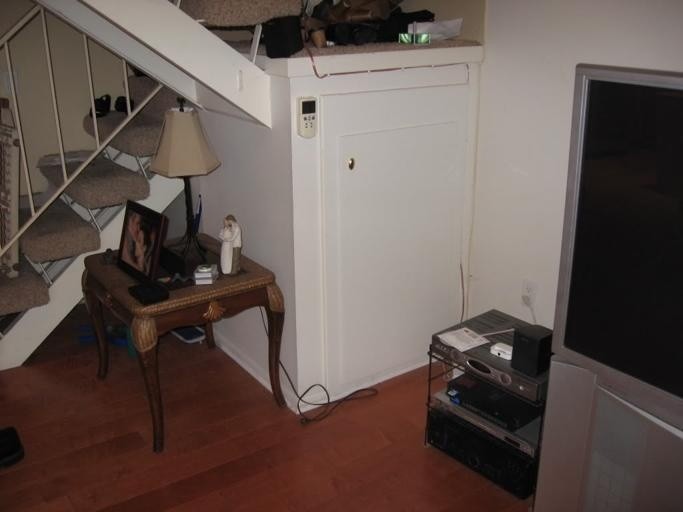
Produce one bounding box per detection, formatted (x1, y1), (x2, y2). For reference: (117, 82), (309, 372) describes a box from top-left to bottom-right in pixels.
(147, 97), (223, 272)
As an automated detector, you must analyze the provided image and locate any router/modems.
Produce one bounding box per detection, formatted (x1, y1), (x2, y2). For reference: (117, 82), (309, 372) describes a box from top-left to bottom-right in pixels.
(490, 341), (513, 361)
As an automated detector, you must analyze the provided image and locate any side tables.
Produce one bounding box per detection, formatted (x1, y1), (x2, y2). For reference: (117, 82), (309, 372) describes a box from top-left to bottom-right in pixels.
(82, 233), (289, 455)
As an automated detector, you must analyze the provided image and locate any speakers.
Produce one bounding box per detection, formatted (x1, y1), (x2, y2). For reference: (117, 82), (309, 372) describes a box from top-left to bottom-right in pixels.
(511, 324), (553, 378)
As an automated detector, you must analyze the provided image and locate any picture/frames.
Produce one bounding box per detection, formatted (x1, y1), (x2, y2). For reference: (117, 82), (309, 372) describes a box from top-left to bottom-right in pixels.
(116, 199), (170, 282)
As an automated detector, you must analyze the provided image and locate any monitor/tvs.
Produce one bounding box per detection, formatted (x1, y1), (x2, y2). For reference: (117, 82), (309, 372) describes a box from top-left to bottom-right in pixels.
(551, 64), (683, 434)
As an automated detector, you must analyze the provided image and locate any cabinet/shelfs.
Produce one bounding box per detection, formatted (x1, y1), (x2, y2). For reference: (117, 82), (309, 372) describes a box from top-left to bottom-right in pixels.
(422, 348), (546, 500)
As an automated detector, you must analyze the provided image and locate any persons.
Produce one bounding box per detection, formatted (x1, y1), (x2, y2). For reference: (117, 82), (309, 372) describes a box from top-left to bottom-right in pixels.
(121, 211), (151, 273)
(217, 213), (243, 275)
